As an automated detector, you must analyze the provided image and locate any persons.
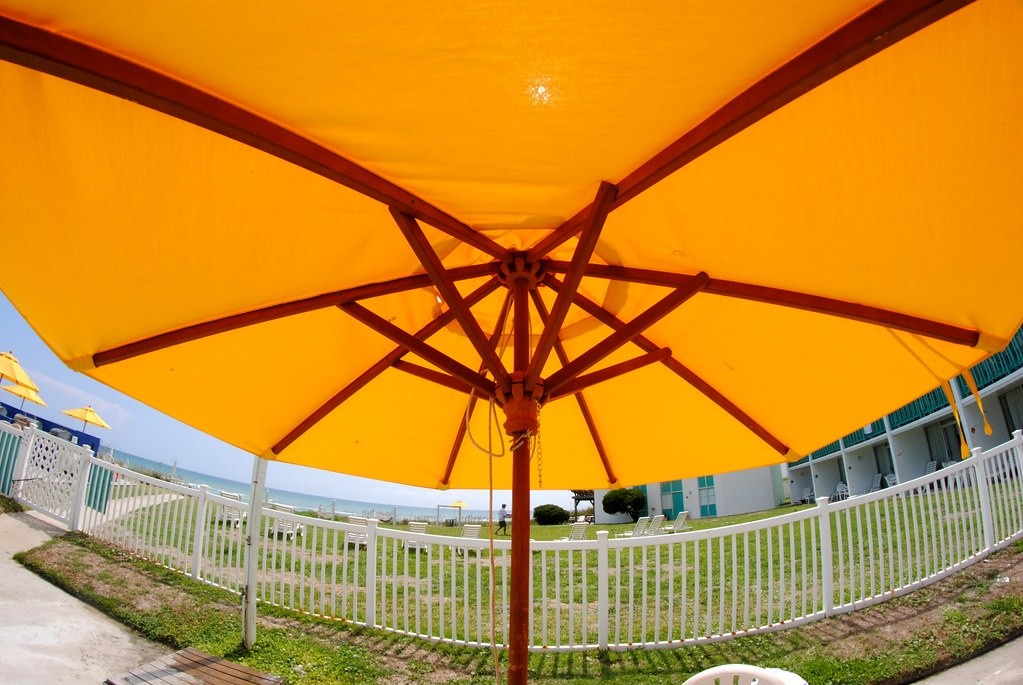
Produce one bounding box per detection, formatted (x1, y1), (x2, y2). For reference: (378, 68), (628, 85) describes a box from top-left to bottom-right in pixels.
(494, 504), (510, 535)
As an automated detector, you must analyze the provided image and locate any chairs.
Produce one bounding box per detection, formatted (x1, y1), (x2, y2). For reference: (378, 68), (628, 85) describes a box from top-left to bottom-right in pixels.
(347, 516), (368, 545)
(793, 447), (1019, 504)
(269, 503), (304, 539)
(554, 521), (590, 551)
(400, 521), (428, 555)
(615, 510), (693, 538)
(217, 489), (248, 528)
(449, 524), (484, 556)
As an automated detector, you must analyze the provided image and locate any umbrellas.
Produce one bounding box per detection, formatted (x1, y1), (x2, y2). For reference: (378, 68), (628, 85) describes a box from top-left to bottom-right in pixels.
(449, 499), (470, 508)
(0, 385), (48, 410)
(0, 350), (39, 392)
(63, 405), (112, 432)
(0, 0), (1023, 685)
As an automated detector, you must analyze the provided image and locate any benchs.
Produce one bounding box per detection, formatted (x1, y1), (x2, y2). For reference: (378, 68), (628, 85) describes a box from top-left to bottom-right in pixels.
(100, 646), (278, 685)
